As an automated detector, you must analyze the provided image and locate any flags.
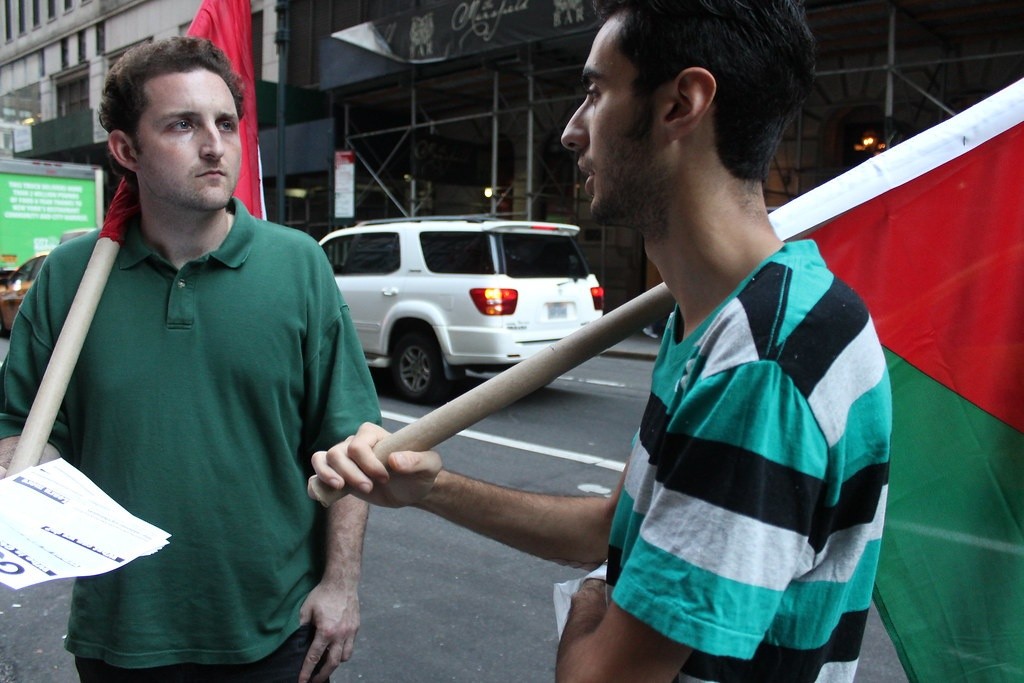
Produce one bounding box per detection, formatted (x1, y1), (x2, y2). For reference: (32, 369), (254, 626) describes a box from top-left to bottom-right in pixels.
(97, 0), (267, 249)
(766, 77), (1024, 683)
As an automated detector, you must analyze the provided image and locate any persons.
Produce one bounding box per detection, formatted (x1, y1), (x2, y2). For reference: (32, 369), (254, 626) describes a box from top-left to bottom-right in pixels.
(305, 1), (893, 683)
(0, 35), (378, 683)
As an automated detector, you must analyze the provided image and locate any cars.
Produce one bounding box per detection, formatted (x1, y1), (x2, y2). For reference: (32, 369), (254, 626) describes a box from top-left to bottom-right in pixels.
(0, 251), (51, 334)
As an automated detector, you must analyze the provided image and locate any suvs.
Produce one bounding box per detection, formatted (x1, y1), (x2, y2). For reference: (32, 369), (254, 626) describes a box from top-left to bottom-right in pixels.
(315, 213), (607, 405)
